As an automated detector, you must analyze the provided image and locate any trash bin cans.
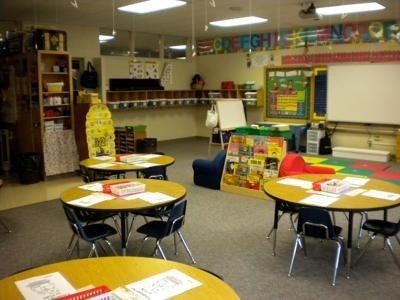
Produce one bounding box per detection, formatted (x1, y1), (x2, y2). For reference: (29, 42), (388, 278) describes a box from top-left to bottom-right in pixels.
(18, 152), (42, 184)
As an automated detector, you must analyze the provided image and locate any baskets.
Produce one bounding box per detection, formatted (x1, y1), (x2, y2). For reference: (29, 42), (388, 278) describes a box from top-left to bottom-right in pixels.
(321, 178), (352, 193)
(51, 284), (124, 300)
(109, 181), (146, 196)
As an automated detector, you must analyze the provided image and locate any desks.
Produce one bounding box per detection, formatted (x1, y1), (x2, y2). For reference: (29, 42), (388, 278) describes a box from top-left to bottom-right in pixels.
(0, 256), (241, 300)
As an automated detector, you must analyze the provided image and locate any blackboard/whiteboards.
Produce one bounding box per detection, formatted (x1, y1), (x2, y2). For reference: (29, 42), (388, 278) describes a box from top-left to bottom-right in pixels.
(327, 63), (400, 125)
(217, 98), (247, 131)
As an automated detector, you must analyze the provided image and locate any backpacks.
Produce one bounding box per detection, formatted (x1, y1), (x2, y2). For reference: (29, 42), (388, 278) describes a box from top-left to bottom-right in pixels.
(319, 135), (333, 155)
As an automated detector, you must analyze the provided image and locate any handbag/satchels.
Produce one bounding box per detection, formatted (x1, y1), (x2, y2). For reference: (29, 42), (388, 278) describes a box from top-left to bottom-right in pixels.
(205, 103), (218, 128)
(79, 62), (99, 89)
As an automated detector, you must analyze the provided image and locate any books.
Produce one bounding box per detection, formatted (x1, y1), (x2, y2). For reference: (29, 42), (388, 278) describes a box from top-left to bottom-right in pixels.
(223, 135), (283, 191)
(312, 178), (351, 194)
(115, 153), (142, 163)
(102, 181), (145, 196)
(50, 285), (123, 299)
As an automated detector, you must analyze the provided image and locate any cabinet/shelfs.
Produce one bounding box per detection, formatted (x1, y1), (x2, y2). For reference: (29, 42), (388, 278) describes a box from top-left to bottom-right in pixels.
(332, 146), (392, 163)
(17, 50), (74, 185)
(106, 88), (265, 111)
(114, 131), (146, 154)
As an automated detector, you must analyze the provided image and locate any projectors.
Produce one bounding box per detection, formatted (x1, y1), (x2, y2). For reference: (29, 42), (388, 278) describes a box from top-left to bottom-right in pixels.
(298, 2), (322, 20)
